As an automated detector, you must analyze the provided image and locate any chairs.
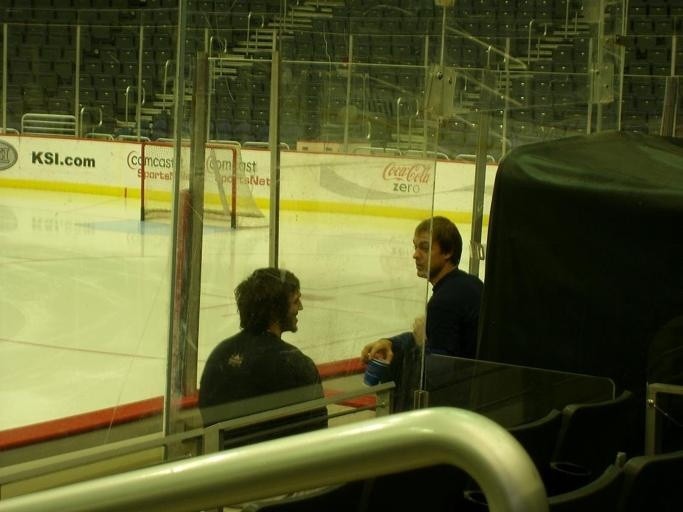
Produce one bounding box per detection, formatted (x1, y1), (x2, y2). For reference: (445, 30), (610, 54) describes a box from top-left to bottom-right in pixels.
(458, 391), (683, 511)
(0, 0), (683, 163)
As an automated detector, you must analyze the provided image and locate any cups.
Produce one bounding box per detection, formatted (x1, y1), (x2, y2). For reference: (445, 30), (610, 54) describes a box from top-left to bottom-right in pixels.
(363, 357), (391, 387)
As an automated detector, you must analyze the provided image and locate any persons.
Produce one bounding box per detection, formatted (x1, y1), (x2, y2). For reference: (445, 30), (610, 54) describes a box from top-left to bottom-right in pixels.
(360, 216), (486, 416)
(192, 268), (329, 454)
